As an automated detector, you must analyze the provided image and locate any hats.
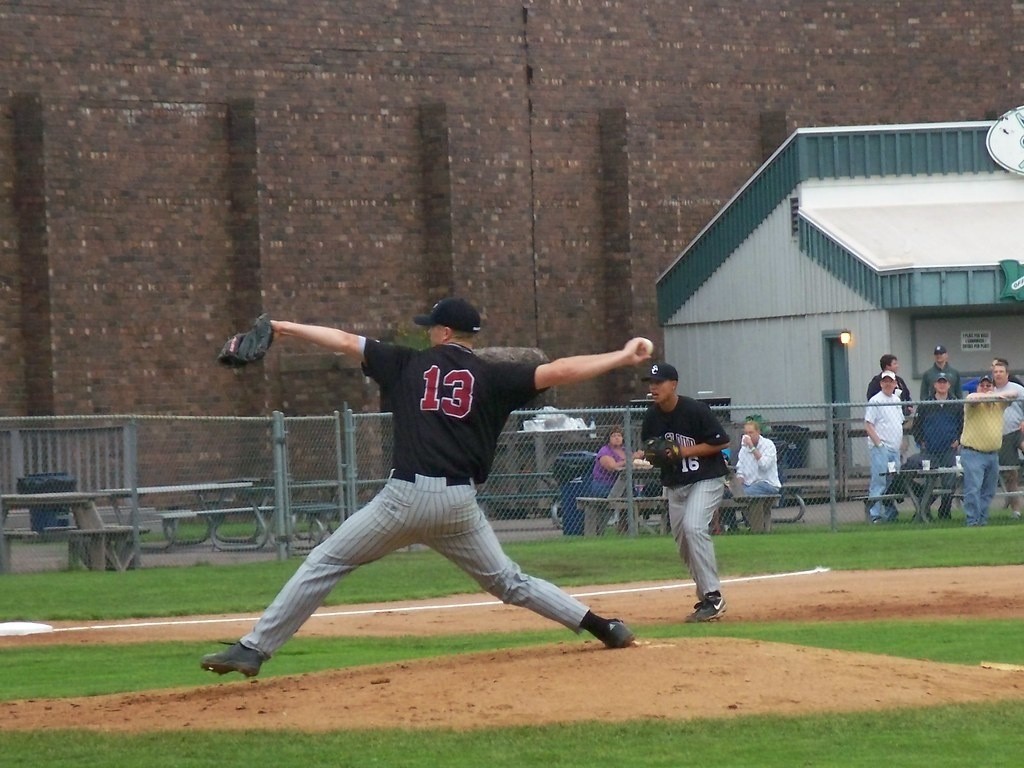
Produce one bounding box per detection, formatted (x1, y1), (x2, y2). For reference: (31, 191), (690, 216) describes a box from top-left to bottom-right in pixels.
(412, 298), (480, 334)
(640, 362), (678, 383)
(934, 345), (946, 355)
(935, 372), (948, 381)
(881, 370), (896, 381)
(979, 376), (993, 384)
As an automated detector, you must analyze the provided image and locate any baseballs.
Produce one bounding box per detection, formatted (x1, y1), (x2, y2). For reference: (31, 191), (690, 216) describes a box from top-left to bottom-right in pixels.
(642, 337), (654, 357)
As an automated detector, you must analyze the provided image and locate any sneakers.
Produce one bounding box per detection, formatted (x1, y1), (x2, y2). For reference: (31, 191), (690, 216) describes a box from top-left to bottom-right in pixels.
(200, 638), (262, 677)
(685, 592), (727, 624)
(602, 619), (636, 649)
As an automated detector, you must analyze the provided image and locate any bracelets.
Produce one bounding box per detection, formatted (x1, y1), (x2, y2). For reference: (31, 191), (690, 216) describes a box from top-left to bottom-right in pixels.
(749, 447), (756, 453)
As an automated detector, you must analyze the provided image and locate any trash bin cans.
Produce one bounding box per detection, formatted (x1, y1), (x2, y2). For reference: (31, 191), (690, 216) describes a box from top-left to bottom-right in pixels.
(771, 424), (812, 468)
(556, 451), (597, 537)
(769, 438), (788, 508)
(16, 471), (77, 532)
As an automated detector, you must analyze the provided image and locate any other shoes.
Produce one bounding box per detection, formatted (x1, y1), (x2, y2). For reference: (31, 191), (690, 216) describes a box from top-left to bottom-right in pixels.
(872, 518), (881, 525)
(1011, 511), (1021, 520)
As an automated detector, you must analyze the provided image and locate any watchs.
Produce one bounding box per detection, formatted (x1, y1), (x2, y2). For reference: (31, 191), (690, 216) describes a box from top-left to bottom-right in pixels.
(877, 443), (883, 447)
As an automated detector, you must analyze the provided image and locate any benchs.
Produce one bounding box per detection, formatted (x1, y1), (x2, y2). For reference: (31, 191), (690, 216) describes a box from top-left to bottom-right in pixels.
(576, 484), (813, 537)
(1, 502), (341, 577)
(851, 488), (1024, 524)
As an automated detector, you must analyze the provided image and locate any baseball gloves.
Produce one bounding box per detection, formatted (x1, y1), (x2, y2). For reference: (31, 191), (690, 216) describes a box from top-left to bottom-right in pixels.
(216, 313), (274, 368)
(644, 437), (682, 468)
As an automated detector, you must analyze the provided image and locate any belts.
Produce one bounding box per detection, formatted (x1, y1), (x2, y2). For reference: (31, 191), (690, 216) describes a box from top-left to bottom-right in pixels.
(392, 471), (471, 486)
(964, 446), (995, 454)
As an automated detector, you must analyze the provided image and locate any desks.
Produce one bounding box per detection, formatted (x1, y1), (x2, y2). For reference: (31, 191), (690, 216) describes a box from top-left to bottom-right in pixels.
(608, 466), (738, 497)
(0, 490), (118, 528)
(880, 464), (1020, 523)
(98, 483), (257, 530)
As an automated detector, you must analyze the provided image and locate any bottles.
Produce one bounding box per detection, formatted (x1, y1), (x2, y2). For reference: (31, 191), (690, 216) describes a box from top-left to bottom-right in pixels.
(635, 485), (646, 498)
(590, 416), (597, 438)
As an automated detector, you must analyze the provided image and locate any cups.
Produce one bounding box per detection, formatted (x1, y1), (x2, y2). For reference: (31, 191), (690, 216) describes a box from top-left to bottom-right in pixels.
(888, 461), (896, 473)
(895, 389), (902, 397)
(956, 455), (962, 468)
(741, 435), (751, 445)
(922, 460), (931, 470)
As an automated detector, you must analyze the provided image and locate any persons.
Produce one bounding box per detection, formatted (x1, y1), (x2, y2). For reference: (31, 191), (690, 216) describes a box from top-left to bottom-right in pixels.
(197, 295), (654, 678)
(592, 424), (646, 531)
(912, 372), (964, 520)
(864, 370), (905, 524)
(640, 364), (733, 623)
(735, 420), (781, 530)
(960, 373), (1019, 526)
(988, 357), (1024, 517)
(920, 345), (963, 400)
(867, 354), (914, 466)
(709, 445), (732, 536)
